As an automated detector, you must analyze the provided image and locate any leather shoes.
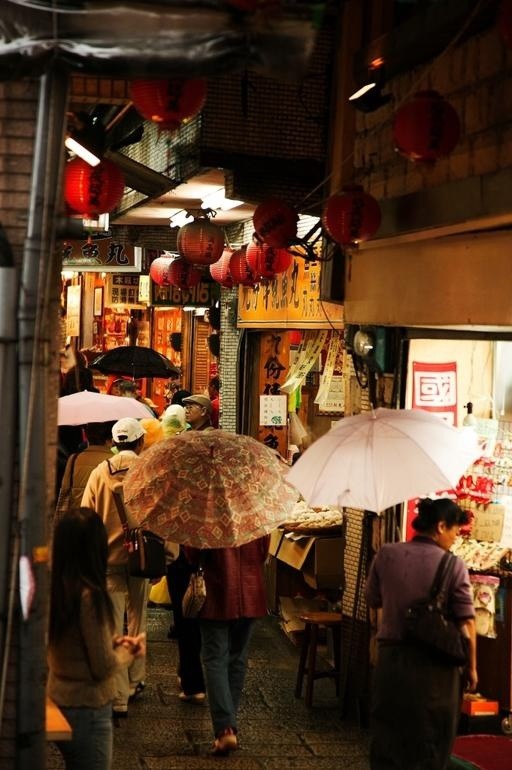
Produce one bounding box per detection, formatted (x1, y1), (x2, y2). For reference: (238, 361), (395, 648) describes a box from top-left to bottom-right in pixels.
(127, 679), (144, 703)
(115, 710), (127, 716)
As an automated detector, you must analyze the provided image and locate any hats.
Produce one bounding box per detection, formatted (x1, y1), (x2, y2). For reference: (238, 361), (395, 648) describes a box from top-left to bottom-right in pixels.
(111, 418), (146, 442)
(121, 380), (139, 389)
(143, 396), (158, 409)
(181, 395), (213, 415)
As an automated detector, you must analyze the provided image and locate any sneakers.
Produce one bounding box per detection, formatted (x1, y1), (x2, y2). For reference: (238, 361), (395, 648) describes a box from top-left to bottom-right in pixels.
(214, 732), (238, 755)
(179, 691), (208, 705)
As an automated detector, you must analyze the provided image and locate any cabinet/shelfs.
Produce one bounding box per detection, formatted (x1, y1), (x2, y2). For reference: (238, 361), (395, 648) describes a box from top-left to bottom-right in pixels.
(468, 570), (512, 712)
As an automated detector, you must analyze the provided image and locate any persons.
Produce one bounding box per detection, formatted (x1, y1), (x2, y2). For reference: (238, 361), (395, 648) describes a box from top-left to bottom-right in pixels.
(46, 506), (148, 770)
(106, 378), (126, 397)
(80, 417), (179, 723)
(363, 497), (477, 769)
(62, 347), (96, 396)
(184, 532), (272, 757)
(208, 374), (219, 428)
(176, 395), (215, 433)
(166, 548), (208, 704)
(119, 380), (138, 398)
(60, 419), (120, 511)
(114, 376), (141, 399)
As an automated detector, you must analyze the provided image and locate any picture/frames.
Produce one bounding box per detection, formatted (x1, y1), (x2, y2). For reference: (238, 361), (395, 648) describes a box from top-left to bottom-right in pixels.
(94, 286), (104, 316)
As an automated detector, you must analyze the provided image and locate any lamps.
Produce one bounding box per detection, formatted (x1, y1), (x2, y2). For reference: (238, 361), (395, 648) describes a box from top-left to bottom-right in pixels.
(65, 110), (106, 167)
(334, 67), (375, 101)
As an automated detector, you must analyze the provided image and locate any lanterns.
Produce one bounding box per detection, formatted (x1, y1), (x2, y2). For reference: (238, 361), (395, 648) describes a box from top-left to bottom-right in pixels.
(390, 87), (461, 164)
(62, 153), (125, 227)
(252, 197), (301, 250)
(241, 239), (294, 283)
(209, 247), (237, 290)
(228, 242), (256, 290)
(148, 248), (191, 298)
(320, 179), (382, 284)
(176, 207), (225, 272)
(163, 257), (201, 301)
(128, 74), (209, 147)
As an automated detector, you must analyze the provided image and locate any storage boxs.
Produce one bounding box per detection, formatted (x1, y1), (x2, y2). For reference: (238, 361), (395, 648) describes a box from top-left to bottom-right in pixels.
(276, 535), (345, 589)
(462, 699), (498, 716)
(269, 530), (286, 557)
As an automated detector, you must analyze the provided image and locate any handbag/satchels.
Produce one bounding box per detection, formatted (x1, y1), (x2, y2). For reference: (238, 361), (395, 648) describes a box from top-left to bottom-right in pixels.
(402, 549), (459, 658)
(180, 545), (208, 623)
(111, 488), (167, 580)
(53, 450), (84, 521)
(447, 555), (472, 666)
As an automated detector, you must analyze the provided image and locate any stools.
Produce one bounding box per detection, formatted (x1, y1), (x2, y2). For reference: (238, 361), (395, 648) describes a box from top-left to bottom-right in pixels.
(295, 614), (342, 709)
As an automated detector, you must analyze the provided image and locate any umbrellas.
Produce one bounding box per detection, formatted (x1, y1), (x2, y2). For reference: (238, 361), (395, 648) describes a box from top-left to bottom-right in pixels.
(279, 398), (484, 546)
(121, 430), (307, 552)
(58, 389), (155, 426)
(87, 345), (182, 385)
